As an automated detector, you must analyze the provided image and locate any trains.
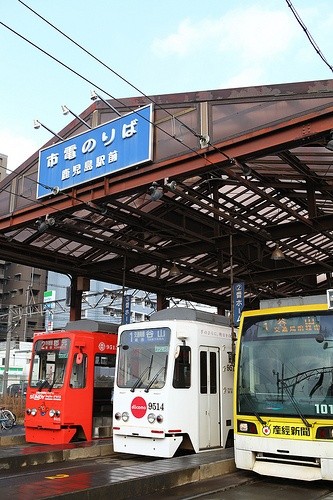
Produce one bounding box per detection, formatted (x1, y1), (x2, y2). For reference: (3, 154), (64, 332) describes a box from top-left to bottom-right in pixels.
(112, 307), (260, 458)
(233, 289), (333, 482)
(24, 319), (123, 445)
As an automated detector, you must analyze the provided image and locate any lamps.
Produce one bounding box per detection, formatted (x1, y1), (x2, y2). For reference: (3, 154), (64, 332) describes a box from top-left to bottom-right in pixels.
(103, 289), (115, 299)
(164, 177), (176, 190)
(325, 131), (333, 151)
(90, 90), (121, 117)
(35, 214), (55, 232)
(271, 188), (284, 261)
(61, 104), (91, 129)
(149, 181), (163, 202)
(33, 118), (64, 141)
(169, 259), (180, 276)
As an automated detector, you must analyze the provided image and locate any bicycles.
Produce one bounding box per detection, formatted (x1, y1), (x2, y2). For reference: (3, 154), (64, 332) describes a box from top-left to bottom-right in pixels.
(0, 406), (15, 431)
